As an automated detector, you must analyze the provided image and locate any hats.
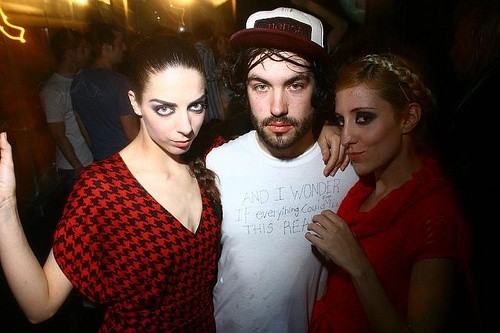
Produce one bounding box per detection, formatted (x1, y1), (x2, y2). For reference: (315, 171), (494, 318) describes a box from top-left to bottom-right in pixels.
(230, 6), (326, 60)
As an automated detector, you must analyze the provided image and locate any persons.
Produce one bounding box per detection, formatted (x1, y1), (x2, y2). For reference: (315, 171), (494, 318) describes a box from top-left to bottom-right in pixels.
(304, 49), (481, 333)
(204, 6), (360, 333)
(38, 28), (93, 213)
(0, 38), (223, 333)
(69, 22), (141, 162)
(192, 24), (226, 122)
